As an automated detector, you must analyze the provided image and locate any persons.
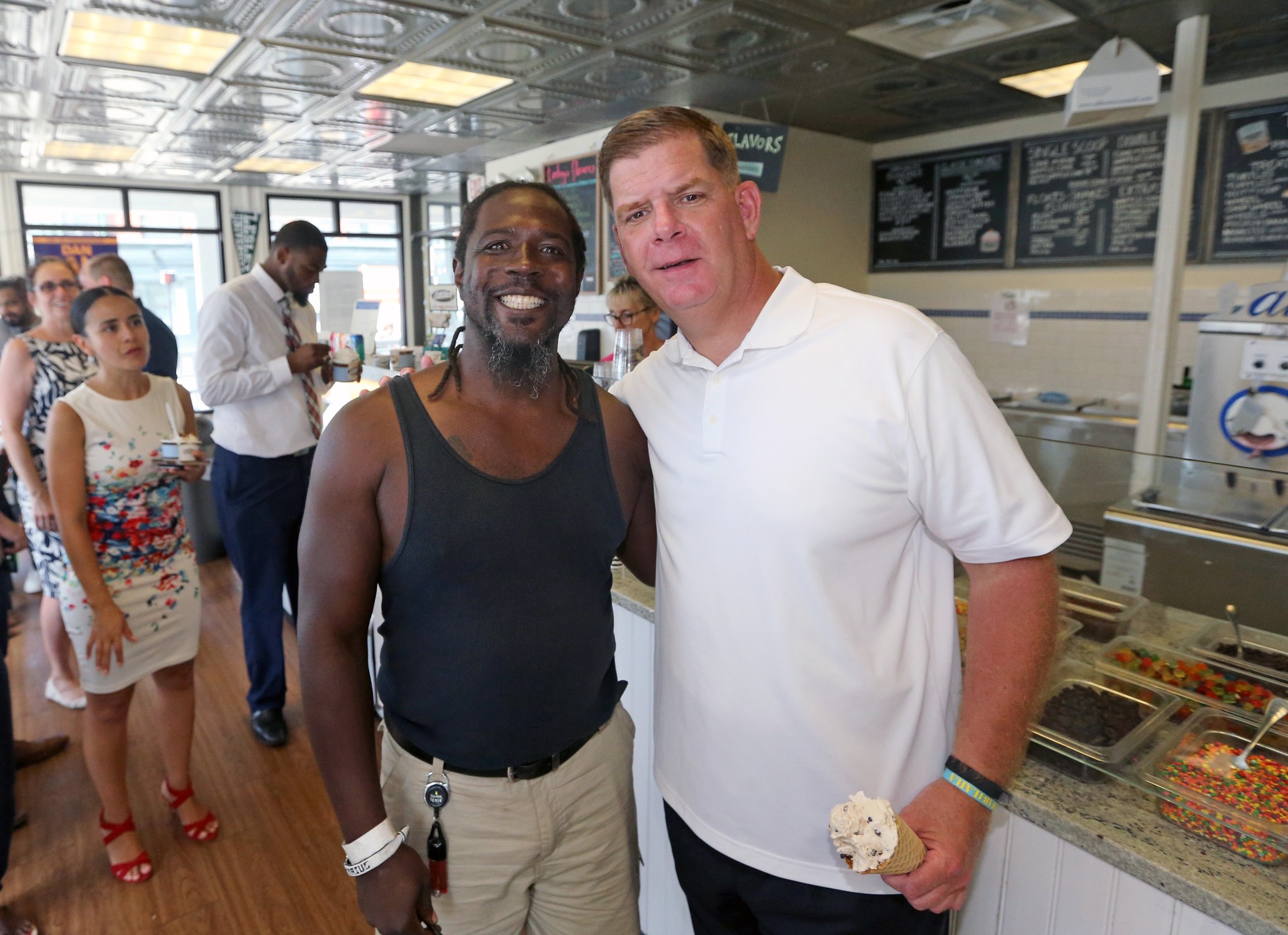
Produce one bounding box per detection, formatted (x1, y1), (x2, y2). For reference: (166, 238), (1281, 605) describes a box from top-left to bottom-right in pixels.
(602, 103), (1073, 935)
(296, 181), (656, 935)
(0, 255), (100, 935)
(77, 252), (177, 382)
(600, 277), (665, 369)
(196, 220), (364, 747)
(43, 286), (219, 883)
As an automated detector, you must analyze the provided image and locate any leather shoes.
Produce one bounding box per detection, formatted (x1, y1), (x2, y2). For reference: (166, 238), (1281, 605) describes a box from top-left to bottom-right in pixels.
(11, 808), (28, 829)
(11, 733), (69, 770)
(248, 703), (288, 746)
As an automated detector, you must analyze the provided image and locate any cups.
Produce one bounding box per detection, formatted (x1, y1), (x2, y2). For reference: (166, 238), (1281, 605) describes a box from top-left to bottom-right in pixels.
(333, 356), (361, 382)
(592, 329), (645, 380)
(160, 440), (202, 462)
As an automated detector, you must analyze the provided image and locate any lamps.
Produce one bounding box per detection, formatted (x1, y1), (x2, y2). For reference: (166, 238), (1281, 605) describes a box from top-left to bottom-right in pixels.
(1062, 0), (1161, 129)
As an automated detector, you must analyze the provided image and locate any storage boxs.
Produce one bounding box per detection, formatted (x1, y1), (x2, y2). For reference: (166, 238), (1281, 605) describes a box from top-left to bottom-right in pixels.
(955, 576), (1288, 867)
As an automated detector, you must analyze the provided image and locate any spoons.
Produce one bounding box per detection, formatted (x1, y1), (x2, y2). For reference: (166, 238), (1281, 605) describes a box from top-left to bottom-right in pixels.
(1225, 604), (1244, 664)
(1210, 697), (1288, 771)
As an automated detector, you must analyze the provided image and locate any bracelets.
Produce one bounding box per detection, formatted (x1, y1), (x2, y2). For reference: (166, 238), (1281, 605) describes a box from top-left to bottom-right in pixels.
(341, 816), (410, 877)
(942, 754), (1012, 812)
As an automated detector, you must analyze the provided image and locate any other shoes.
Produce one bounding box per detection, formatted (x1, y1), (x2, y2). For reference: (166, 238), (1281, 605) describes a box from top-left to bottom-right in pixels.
(23, 568), (43, 594)
(45, 676), (87, 709)
(0, 906), (39, 935)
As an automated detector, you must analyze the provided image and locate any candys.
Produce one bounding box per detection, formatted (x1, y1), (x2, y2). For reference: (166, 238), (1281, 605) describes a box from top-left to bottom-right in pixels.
(1099, 647), (1288, 750)
(1156, 733), (1288, 865)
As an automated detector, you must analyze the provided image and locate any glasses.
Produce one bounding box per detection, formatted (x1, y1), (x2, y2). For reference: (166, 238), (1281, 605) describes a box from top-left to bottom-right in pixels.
(604, 304), (652, 326)
(35, 278), (79, 294)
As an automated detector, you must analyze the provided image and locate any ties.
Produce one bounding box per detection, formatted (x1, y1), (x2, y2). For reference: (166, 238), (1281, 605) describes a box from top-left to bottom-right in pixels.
(277, 297), (322, 440)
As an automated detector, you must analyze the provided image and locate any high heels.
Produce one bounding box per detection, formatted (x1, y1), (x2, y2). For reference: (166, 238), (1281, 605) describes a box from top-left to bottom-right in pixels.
(98, 803), (154, 883)
(162, 777), (219, 843)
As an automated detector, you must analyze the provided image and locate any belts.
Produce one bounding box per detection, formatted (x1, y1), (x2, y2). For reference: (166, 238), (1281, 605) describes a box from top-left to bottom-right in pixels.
(383, 679), (628, 781)
(291, 446), (316, 457)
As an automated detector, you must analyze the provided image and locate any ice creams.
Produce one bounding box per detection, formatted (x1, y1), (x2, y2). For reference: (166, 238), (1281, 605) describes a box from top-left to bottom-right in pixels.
(829, 790), (927, 875)
(160, 432), (202, 462)
(332, 346), (360, 382)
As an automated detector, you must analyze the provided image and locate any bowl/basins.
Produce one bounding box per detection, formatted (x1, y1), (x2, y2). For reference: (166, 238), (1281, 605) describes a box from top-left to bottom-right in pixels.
(1133, 708), (1288, 867)
(1023, 659), (1183, 783)
(1091, 634), (1288, 752)
(1055, 575), (1149, 643)
(1184, 623), (1287, 682)
(954, 585), (1084, 670)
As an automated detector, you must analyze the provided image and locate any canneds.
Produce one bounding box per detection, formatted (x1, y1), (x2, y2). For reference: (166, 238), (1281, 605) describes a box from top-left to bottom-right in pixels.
(349, 334), (365, 363)
